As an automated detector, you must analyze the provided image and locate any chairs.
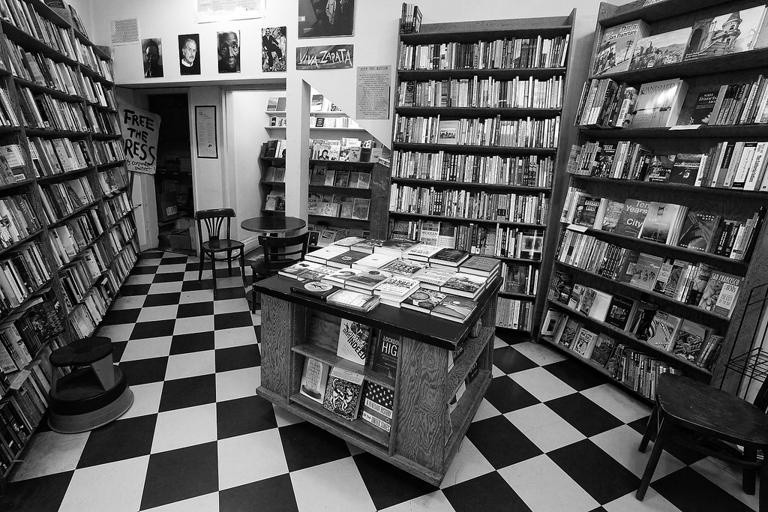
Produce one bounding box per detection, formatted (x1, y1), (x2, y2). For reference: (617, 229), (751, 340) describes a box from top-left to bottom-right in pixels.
(251, 232), (309, 313)
(195, 208), (246, 288)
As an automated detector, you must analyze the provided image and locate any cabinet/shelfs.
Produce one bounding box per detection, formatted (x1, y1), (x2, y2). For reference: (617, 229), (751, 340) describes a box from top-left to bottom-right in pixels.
(387, 7), (577, 338)
(533, 0), (768, 435)
(250, 236), (504, 490)
(258, 94), (392, 250)
(1, 0), (143, 484)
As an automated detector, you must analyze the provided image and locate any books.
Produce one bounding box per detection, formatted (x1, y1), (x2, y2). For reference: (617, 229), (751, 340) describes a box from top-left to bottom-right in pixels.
(0, 0), (140, 477)
(540, 4), (768, 404)
(263, 34), (542, 433)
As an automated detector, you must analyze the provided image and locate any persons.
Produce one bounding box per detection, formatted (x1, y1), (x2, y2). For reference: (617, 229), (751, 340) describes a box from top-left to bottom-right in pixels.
(262, 28), (282, 70)
(180, 38), (200, 74)
(143, 41), (164, 78)
(218, 32), (240, 73)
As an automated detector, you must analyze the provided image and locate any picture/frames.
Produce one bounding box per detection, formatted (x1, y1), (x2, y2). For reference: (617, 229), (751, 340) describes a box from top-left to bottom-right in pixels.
(195, 105), (218, 158)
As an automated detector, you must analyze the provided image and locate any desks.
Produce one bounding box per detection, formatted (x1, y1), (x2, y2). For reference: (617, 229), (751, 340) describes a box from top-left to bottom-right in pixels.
(241, 216), (306, 269)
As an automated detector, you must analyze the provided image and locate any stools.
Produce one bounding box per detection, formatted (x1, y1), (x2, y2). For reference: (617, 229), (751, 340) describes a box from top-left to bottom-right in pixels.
(47, 336), (134, 434)
(635, 372), (768, 502)
